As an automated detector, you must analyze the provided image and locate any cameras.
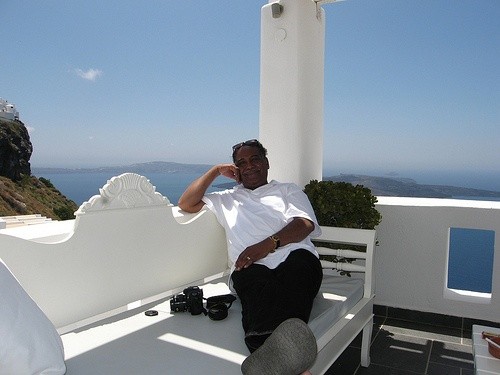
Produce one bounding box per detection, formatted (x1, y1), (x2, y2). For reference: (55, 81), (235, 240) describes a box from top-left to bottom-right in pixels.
(170, 286), (204, 315)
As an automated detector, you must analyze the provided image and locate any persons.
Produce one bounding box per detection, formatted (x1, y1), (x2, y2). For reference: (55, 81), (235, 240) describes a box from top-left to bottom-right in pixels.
(179, 139), (324, 375)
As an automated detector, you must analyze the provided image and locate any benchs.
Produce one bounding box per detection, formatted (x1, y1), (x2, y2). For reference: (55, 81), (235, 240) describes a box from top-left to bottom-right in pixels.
(0, 172), (376, 375)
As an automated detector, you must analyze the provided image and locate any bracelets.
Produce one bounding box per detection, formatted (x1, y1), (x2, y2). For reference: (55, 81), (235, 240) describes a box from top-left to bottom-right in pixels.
(260, 240), (275, 253)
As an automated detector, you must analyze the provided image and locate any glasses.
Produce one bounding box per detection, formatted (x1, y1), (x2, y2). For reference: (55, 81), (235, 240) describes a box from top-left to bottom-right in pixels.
(232, 139), (265, 163)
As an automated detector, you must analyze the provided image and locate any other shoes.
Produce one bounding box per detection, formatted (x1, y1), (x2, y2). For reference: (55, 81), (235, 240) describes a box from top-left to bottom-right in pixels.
(241, 318), (317, 375)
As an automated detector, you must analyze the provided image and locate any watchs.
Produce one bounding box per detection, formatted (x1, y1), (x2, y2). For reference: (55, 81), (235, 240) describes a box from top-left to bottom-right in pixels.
(269, 234), (281, 249)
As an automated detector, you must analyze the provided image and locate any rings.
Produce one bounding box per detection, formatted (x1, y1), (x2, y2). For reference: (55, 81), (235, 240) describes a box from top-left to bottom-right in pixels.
(246, 256), (251, 260)
